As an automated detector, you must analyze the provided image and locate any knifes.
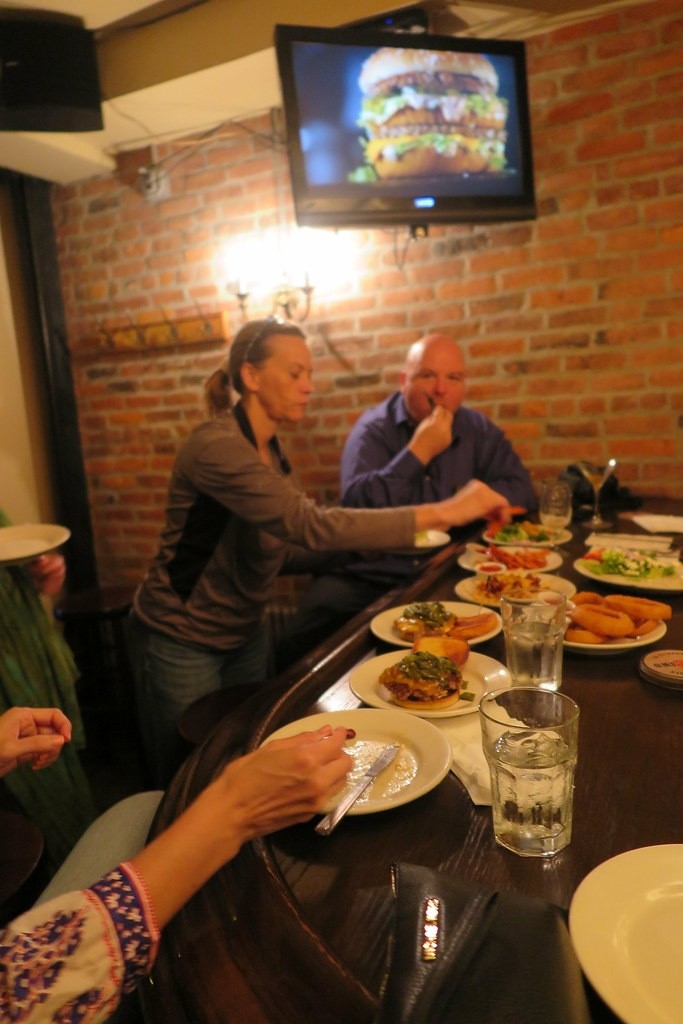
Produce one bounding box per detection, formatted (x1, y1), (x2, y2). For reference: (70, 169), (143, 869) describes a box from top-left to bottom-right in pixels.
(316, 745), (401, 835)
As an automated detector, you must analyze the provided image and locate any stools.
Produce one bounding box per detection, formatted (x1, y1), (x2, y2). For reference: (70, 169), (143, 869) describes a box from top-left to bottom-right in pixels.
(171, 681), (260, 745)
(53, 585), (137, 734)
(0, 811), (45, 911)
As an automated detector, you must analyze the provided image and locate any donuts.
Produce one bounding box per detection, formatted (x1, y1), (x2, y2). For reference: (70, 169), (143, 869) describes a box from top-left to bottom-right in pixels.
(565, 591), (671, 644)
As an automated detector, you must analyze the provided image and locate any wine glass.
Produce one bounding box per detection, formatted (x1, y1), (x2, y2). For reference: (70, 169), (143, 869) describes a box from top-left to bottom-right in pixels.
(539, 480), (572, 559)
(577, 455), (617, 529)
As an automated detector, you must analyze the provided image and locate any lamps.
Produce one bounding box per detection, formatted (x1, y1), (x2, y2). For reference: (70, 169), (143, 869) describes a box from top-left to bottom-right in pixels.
(235, 265), (315, 326)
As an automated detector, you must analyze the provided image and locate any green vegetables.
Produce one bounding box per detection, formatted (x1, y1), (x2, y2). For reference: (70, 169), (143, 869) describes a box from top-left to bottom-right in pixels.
(401, 601), (476, 703)
(493, 524), (550, 542)
(582, 546), (674, 577)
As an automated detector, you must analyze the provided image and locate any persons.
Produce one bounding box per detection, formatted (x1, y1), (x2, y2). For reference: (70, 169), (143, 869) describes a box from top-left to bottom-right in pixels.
(127, 319), (511, 790)
(340, 334), (539, 604)
(0, 725), (354, 1024)
(0, 512), (90, 881)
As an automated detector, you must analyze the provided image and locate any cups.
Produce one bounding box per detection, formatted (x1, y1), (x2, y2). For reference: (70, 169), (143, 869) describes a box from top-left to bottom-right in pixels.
(478, 686), (578, 858)
(502, 588), (565, 692)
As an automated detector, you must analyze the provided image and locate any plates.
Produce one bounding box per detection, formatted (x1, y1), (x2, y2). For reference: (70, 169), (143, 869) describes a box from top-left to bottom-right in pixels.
(482, 524), (573, 547)
(369, 600), (502, 646)
(573, 553), (683, 590)
(1, 524), (71, 565)
(567, 842), (683, 1024)
(257, 708), (451, 815)
(563, 618), (667, 655)
(458, 547), (563, 573)
(350, 648), (510, 719)
(455, 572), (576, 608)
(414, 529), (450, 555)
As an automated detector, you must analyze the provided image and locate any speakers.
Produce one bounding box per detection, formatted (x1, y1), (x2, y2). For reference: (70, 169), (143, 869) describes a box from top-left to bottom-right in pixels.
(0, 22), (104, 132)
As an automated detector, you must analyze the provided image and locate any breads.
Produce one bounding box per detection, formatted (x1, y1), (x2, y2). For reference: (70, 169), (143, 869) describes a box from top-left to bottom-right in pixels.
(380, 613), (499, 708)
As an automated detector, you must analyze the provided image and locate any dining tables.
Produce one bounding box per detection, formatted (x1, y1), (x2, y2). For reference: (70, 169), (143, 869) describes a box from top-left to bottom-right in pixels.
(140, 495), (683, 1024)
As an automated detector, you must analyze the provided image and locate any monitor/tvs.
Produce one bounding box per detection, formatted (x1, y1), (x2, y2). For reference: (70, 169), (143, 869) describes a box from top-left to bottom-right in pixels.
(272, 25), (538, 226)
